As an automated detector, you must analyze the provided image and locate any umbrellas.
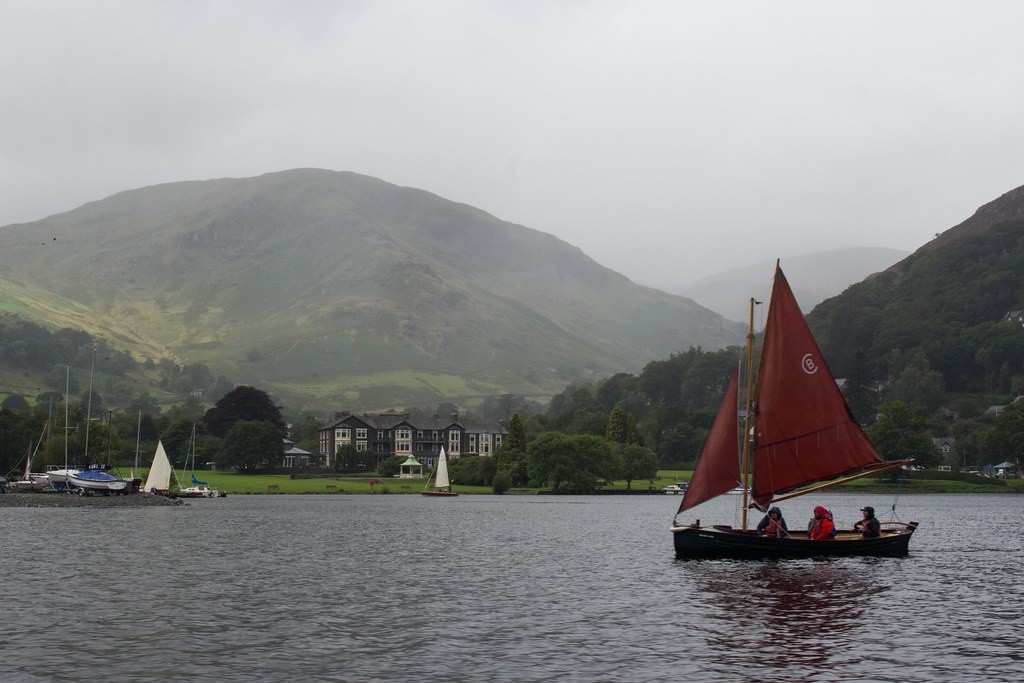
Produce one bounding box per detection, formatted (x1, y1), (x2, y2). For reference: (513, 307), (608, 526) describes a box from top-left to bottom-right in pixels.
(993, 462), (1015, 480)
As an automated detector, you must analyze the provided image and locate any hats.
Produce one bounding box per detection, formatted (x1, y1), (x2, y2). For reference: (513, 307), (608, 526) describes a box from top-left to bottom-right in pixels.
(860, 507), (874, 511)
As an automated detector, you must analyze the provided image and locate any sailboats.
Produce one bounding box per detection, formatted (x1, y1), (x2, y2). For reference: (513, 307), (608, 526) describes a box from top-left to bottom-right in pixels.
(669, 258), (921, 558)
(0, 344), (227, 499)
(421, 445), (459, 496)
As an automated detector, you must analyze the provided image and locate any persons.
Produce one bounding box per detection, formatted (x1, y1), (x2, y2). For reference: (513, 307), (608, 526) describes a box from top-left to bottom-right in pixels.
(854, 507), (881, 538)
(369, 478), (375, 490)
(209, 489), (226, 498)
(149, 487), (176, 500)
(756, 507), (788, 538)
(1003, 469), (1021, 478)
(808, 506), (836, 540)
(288, 464), (303, 474)
(4, 486), (13, 493)
